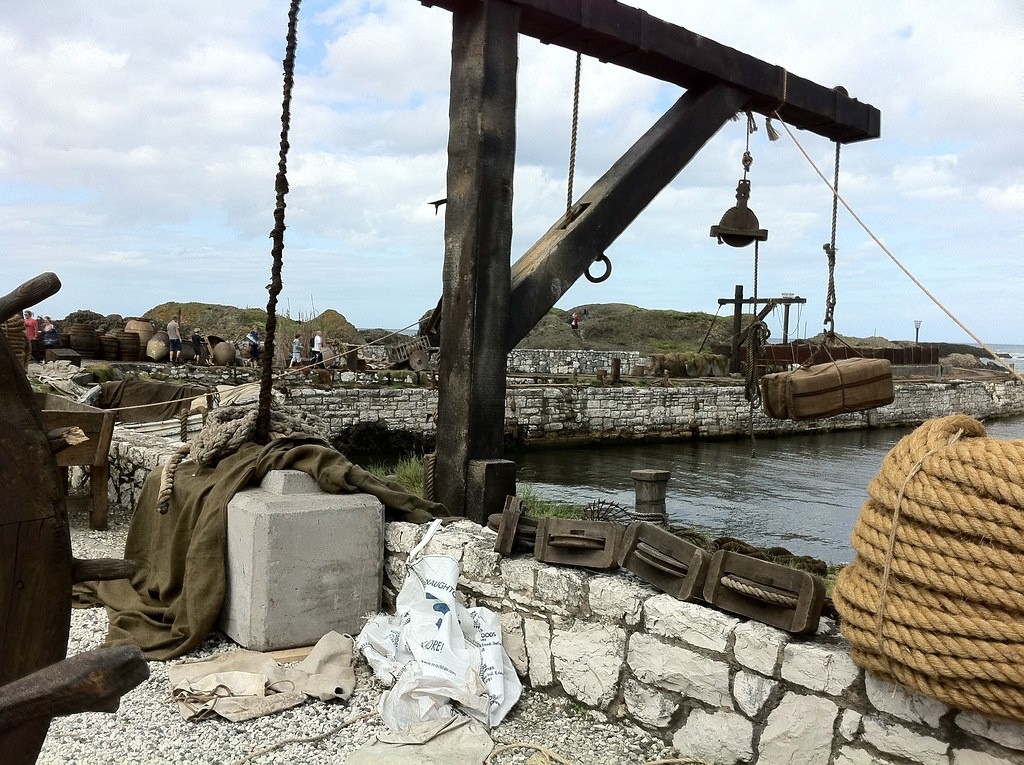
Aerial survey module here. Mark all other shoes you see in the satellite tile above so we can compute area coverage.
[175,357,180,363]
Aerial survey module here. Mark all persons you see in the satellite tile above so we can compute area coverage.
[39,316,60,351]
[24,310,38,359]
[245,324,260,368]
[166,316,183,364]
[567,313,579,329]
[190,327,204,363]
[309,330,330,369]
[289,332,304,368]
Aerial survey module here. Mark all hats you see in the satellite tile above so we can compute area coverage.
[194,328,200,332]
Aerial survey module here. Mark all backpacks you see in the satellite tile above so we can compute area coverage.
[310,337,314,347]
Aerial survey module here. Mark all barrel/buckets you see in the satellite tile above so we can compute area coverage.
[116,332,141,361]
[145,331,170,363]
[69,322,96,359]
[98,336,118,361]
[124,318,154,361]
[213,342,236,366]
